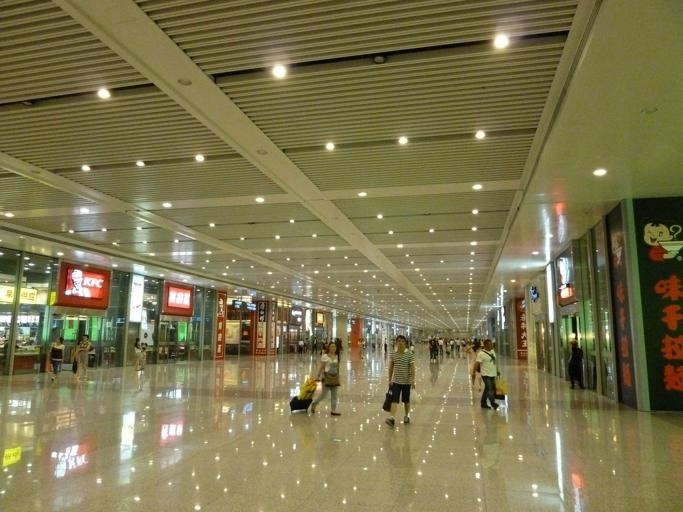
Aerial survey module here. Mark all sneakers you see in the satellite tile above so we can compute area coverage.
[404,417,409,423]
[385,417,394,427]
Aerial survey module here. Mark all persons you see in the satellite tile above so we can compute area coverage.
[310,341,341,416]
[567,341,585,389]
[308,416,338,484]
[473,409,506,495]
[46,337,64,383]
[65,269,91,299]
[382,428,416,508]
[73,334,91,380]
[290,336,485,365]
[139,347,146,370]
[384,334,415,428]
[133,337,141,371]
[471,338,500,409]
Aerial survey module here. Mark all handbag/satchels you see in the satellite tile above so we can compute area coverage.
[324,374,339,386]
[298,384,316,400]
[383,388,393,411]
[495,375,509,400]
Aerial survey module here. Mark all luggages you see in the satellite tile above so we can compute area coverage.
[290,380,320,412]
